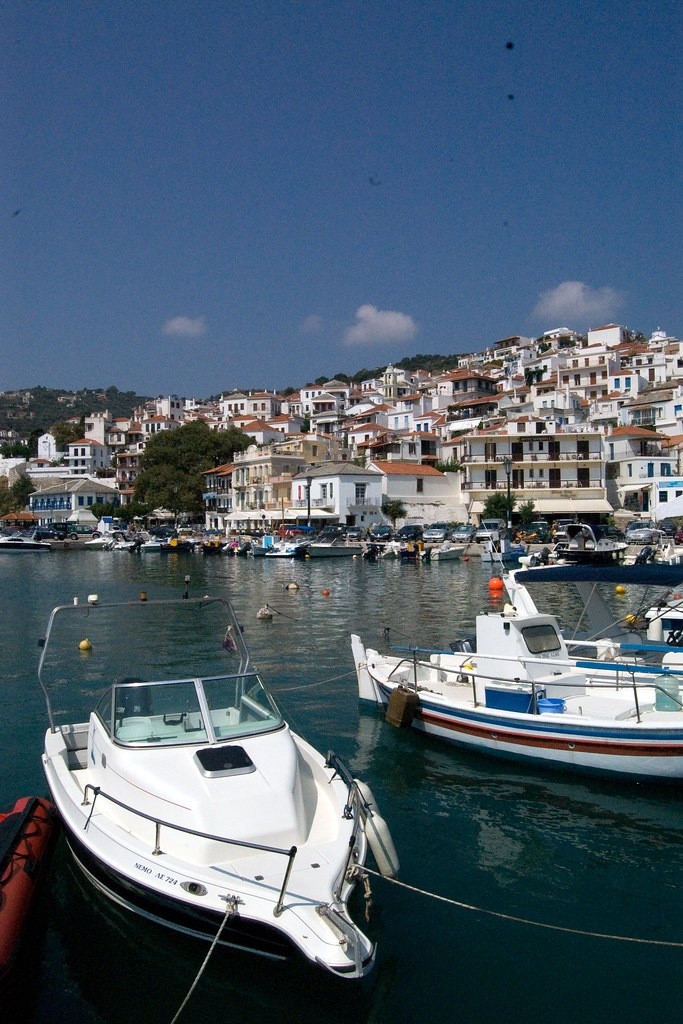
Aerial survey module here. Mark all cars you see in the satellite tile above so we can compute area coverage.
[421,522,454,543]
[369,524,395,542]
[394,525,424,542]
[342,525,368,542]
[452,524,477,543]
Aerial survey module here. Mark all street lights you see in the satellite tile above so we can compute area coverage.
[502,459,515,541]
[304,466,314,527]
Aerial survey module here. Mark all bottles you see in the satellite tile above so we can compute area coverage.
[656,667,681,711]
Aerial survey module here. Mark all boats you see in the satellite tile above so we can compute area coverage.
[348,563,683,788]
[0,518,683,574]
[643,583,683,650]
[37,595,398,981]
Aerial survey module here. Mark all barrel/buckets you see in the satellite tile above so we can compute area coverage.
[537,698,567,714]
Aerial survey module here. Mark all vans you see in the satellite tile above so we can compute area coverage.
[474,519,507,544]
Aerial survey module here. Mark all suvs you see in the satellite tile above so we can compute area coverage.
[29,525,66,542]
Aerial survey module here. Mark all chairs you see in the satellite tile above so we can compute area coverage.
[569,539,579,549]
[117,717,154,732]
[585,540,595,549]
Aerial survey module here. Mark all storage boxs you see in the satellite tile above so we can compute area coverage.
[484,680,546,714]
